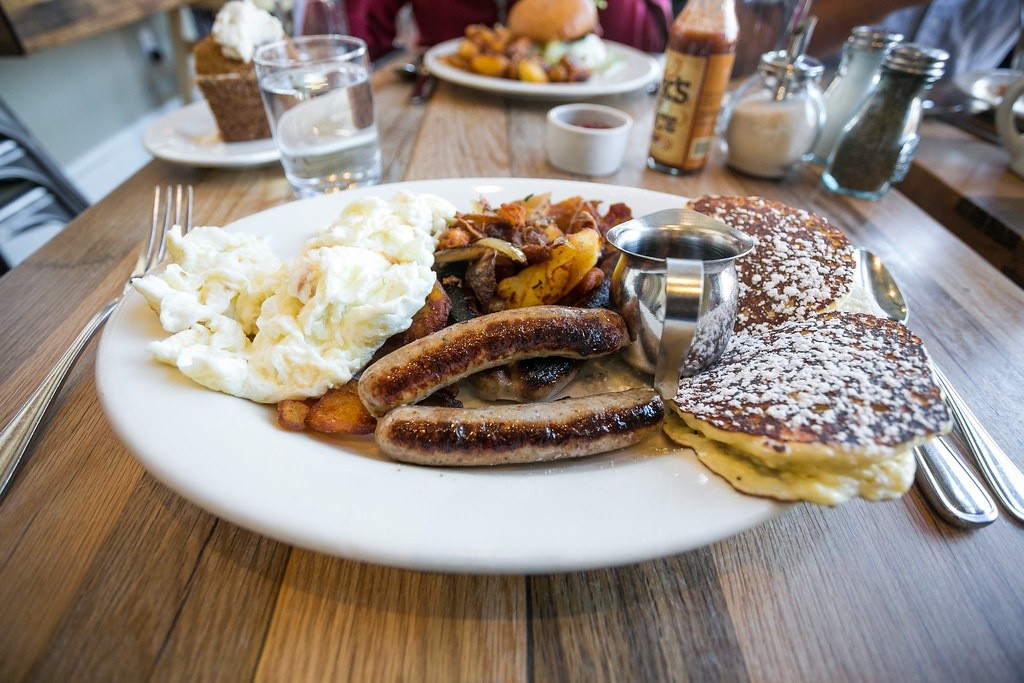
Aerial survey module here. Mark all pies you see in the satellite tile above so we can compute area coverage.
[661,194,954,507]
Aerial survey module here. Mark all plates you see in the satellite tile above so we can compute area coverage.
[140,99,281,169]
[91,176,885,577]
[421,34,660,100]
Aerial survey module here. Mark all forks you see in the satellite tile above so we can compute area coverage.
[0,183,195,507]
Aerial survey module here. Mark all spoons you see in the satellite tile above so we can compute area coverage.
[848,247,1024,523]
[392,54,426,79]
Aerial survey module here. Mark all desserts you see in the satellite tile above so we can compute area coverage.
[194,0,305,144]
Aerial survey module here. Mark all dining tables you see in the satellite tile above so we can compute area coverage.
[0,0,1024,683]
[901,94,1024,291]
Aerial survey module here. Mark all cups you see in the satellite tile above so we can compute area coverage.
[994,77,1024,178]
[603,206,754,401]
[248,0,350,80]
[543,103,633,178]
[250,32,383,200]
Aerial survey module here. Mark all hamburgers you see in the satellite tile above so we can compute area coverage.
[506,0,609,81]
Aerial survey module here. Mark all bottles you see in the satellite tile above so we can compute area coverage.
[719,49,827,180]
[820,41,949,203]
[811,24,905,169]
[645,0,742,177]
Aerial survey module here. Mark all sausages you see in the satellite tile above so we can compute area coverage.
[374,387,667,467]
[438,250,619,403]
[356,306,630,419]
